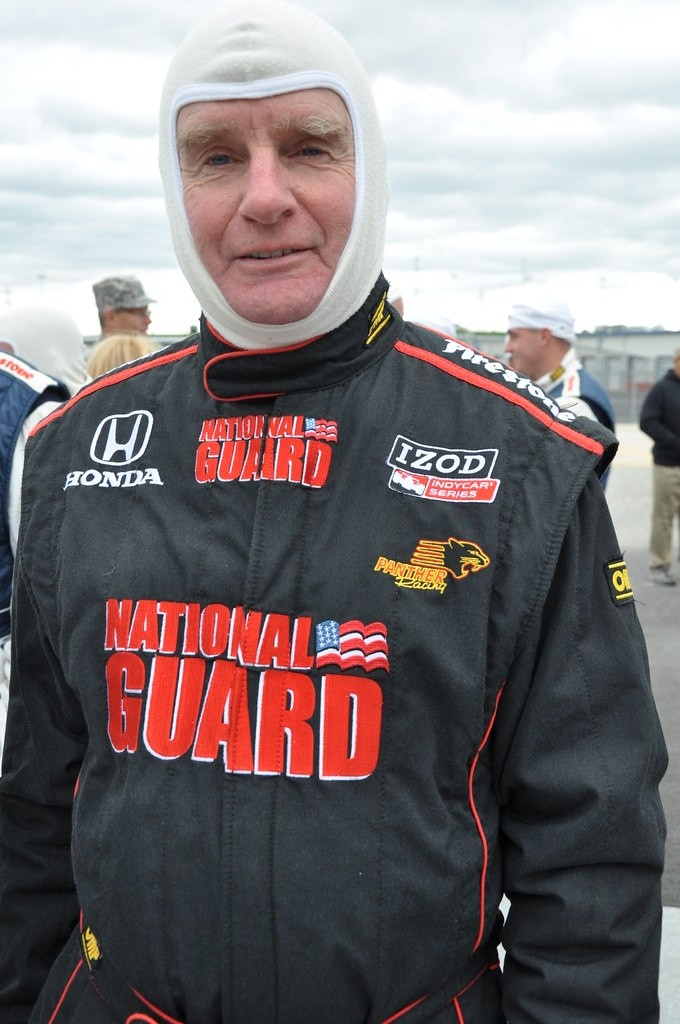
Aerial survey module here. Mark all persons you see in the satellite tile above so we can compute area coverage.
[504,301,615,491]
[0,350,73,747]
[640,348,680,585]
[0,2,670,1024]
[86,276,156,378]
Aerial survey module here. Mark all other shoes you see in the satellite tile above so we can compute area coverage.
[648,565,677,585]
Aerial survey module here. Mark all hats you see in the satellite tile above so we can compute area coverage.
[93,274,157,316]
[508,293,576,344]
